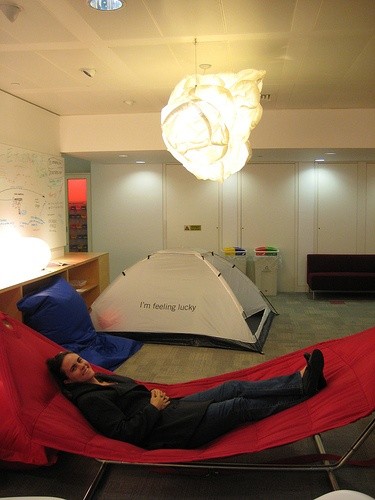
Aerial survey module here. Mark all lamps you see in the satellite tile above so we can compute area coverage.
[161,38,267,182]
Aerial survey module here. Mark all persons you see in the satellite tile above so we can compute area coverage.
[46,349,328,451]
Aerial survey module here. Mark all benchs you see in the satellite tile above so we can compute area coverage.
[307,254,375,301]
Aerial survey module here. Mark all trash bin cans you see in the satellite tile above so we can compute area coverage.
[254,246,279,296]
[221,247,246,275]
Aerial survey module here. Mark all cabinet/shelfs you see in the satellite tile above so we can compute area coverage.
[0,253,110,323]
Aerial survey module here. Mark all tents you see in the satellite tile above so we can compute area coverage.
[86,247,279,357]
